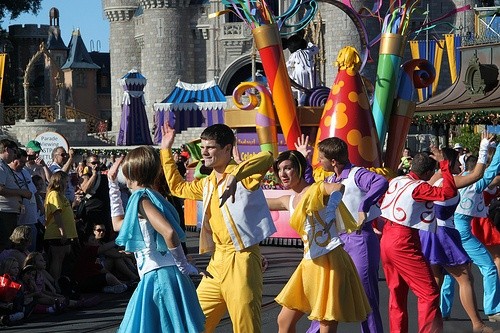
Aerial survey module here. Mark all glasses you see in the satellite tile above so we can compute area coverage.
[95,229,106,232]
[9,147,17,154]
[56,153,67,157]
[89,162,99,165]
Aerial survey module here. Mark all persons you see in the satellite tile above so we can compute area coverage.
[106,145,214,333]
[294,134,389,333]
[371,128,500,333]
[266,149,373,333]
[159,121,277,333]
[0,137,190,321]
[397,147,412,177]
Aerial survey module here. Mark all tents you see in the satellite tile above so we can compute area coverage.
[152,79,227,145]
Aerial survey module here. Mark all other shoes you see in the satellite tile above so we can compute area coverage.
[104,284,125,293]
[79,295,99,309]
[488,313,500,323]
[53,299,63,314]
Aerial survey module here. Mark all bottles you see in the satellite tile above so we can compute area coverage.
[103,284,129,293]
[1,311,27,323]
[47,303,61,312]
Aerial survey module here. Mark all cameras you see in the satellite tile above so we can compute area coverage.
[95,163,107,171]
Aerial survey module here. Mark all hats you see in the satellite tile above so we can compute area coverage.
[26,140,44,153]
[453,143,463,149]
